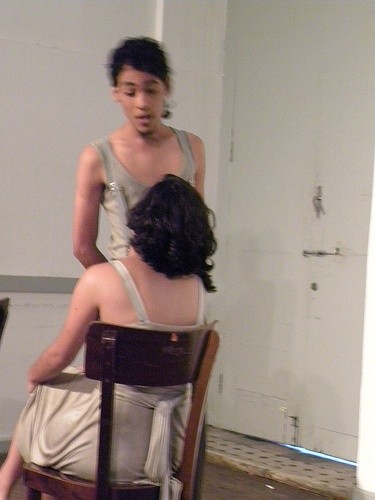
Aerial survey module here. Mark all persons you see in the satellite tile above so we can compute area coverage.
[72,36,207,270]
[0,174,218,500]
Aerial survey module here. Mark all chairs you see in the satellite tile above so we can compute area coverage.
[21,319,221,500]
